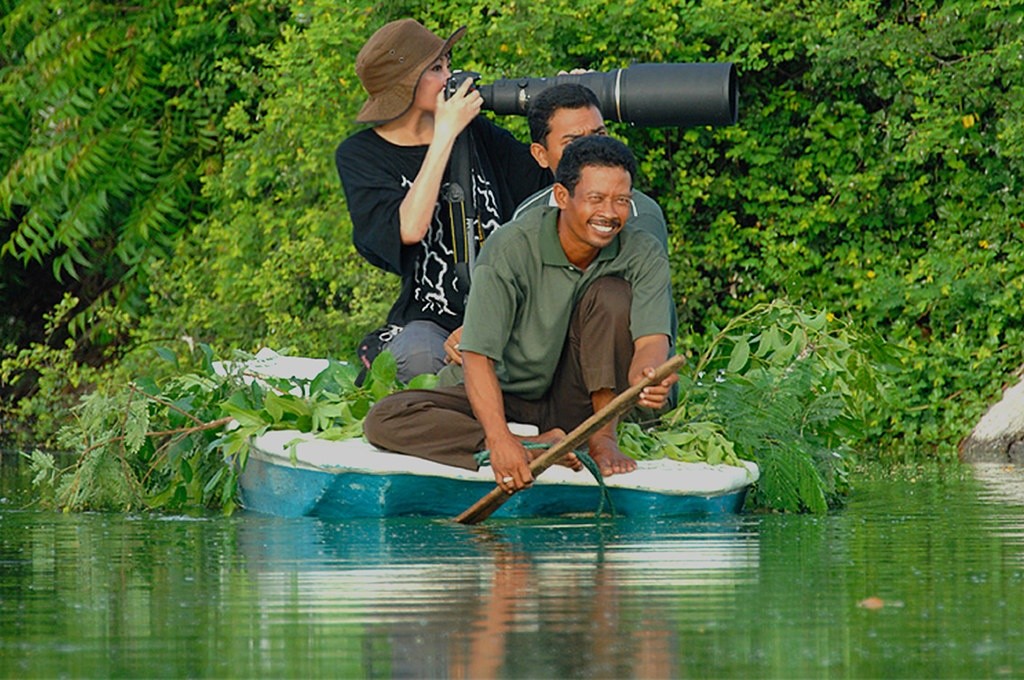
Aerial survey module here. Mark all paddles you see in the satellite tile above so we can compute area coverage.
[455,352,688,525]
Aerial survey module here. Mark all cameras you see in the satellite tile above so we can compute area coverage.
[444,62,742,128]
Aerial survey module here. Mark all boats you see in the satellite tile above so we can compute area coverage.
[211,347,761,521]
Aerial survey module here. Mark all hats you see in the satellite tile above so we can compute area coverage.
[352,19,467,123]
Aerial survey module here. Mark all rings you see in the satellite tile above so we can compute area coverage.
[502,477,513,483]
[452,344,460,351]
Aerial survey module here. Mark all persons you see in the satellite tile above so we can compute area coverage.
[336,19,596,388]
[363,134,678,496]
[436,83,678,423]
